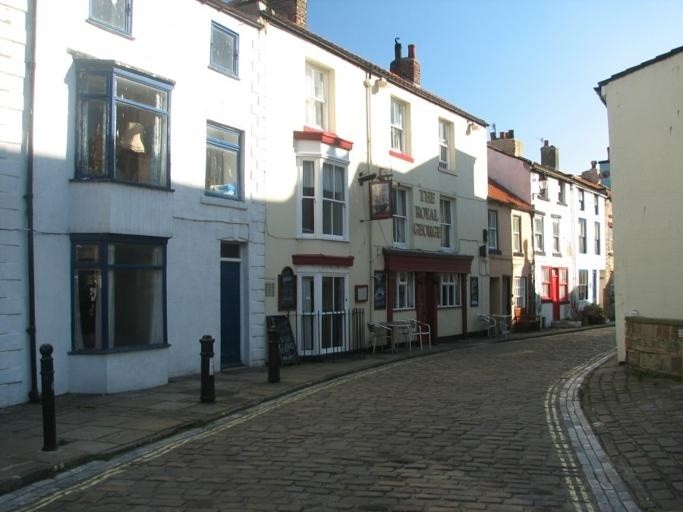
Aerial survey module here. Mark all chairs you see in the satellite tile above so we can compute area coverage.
[478,313,497,337]
[369,319,431,353]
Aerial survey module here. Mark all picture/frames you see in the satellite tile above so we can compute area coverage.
[368,178,393,220]
[355,285,368,302]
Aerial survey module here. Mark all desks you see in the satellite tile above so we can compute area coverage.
[515,315,541,331]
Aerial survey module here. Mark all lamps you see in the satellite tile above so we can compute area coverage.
[470,123,479,130]
[378,77,390,88]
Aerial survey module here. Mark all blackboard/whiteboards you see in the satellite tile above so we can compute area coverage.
[266,315,299,365]
